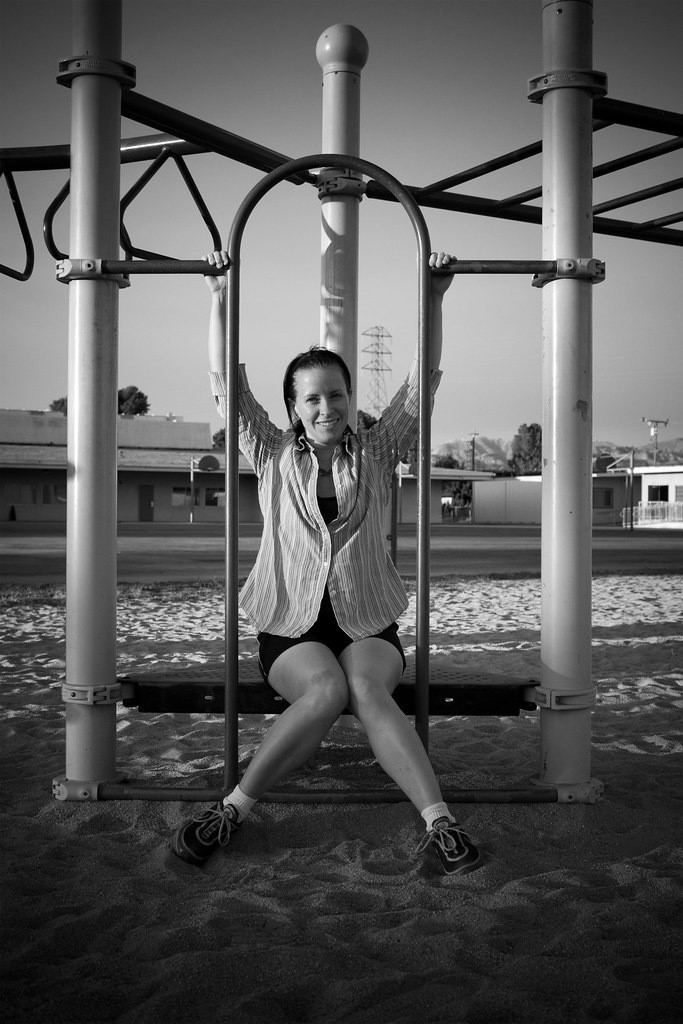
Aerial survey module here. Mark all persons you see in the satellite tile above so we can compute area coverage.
[173,251,484,876]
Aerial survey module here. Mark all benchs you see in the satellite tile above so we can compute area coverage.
[122,662,541,720]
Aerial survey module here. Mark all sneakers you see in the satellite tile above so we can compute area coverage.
[172,800,246,863]
[416,817,480,875]
